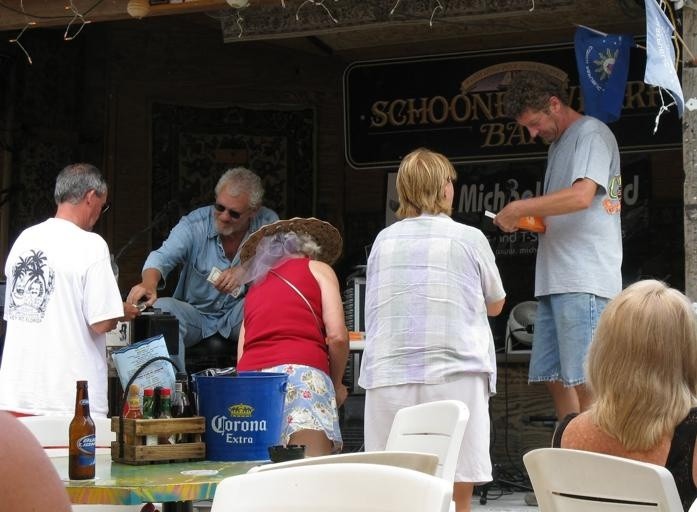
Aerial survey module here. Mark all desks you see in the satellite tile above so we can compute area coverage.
[36,457,278,512]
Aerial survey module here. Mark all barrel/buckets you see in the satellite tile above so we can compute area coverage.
[190,369,289,463]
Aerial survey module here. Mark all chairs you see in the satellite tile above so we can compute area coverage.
[20,418,112,455]
[212,464,450,512]
[383,401,470,512]
[245,450,439,474]
[524,447,682,512]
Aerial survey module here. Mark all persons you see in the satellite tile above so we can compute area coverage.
[356,149,507,511]
[126,167,281,373]
[494,71,623,419]
[0,164,126,420]
[235,217,350,461]
[552,278,697,508]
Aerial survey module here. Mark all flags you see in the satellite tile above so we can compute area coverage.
[571,19,637,128]
[643,0,691,122]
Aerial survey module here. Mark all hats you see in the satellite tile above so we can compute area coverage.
[240,217,343,270]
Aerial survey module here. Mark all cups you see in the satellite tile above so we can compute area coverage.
[268,444,307,465]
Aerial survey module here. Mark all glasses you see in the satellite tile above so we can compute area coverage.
[215,203,253,218]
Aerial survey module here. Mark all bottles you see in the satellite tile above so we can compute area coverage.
[176,372,194,443]
[68,381,96,479]
[124,386,145,446]
[141,389,153,419]
[158,389,175,444]
[172,383,192,443]
[146,387,164,445]
